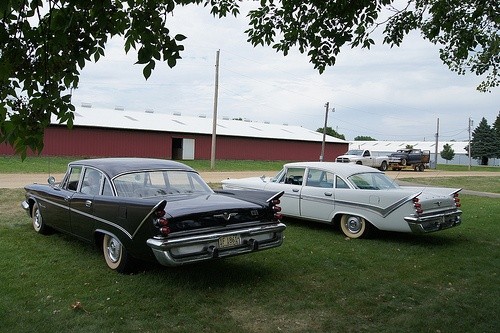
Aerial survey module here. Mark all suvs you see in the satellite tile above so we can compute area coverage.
[388,148,430,171]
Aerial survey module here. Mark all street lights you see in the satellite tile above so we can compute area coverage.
[319,108,336,161]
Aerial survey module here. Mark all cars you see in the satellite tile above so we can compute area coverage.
[21,158,287,274]
[220,162,464,239]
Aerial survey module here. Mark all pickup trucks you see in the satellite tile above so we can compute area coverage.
[335,149,389,170]
